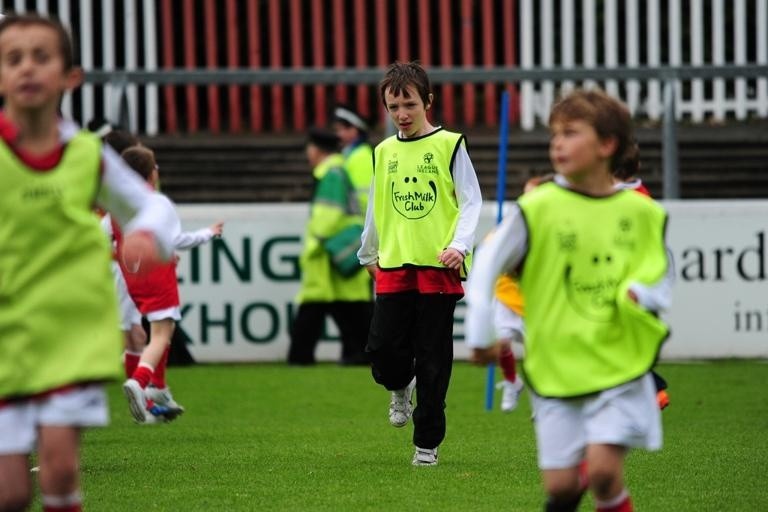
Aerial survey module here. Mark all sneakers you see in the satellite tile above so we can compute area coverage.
[123,378,184,424]
[500,376,524,412]
[655,389,670,410]
[389,375,417,427]
[413,446,438,466]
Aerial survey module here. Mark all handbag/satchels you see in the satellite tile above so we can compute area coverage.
[327,224,364,276]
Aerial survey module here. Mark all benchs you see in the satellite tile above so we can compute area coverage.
[144,118,765,205]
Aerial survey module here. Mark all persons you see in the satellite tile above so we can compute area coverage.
[1,17,180,512]
[465,90,669,512]
[85,116,224,426]
[287,106,373,364]
[357,64,482,465]
[483,141,669,411]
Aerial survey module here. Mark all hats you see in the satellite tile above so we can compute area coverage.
[333,103,373,133]
